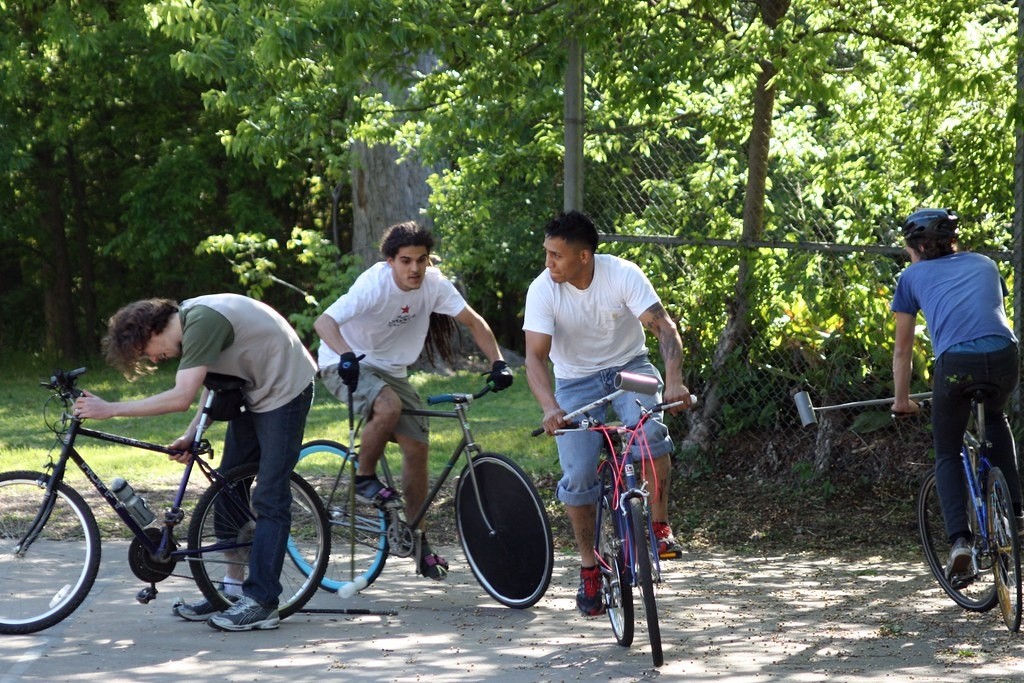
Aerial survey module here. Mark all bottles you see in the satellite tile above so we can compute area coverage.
[110,477,157,528]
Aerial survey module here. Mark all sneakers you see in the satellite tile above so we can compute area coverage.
[397,530,448,580]
[173,583,240,620]
[1016,511,1024,536]
[207,594,280,630]
[944,537,972,580]
[576,565,606,615]
[353,477,403,508]
[652,518,682,558]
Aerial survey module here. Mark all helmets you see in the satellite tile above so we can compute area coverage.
[902,209,959,239]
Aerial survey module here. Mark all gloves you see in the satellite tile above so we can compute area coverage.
[480,361,513,393]
[338,353,365,393]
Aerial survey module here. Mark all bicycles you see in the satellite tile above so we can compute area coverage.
[0,366,331,634]
[891,383,1022,632]
[555,395,698,667]
[287,360,554,611]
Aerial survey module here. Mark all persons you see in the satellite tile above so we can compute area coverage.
[313,221,514,581]
[524,212,692,616]
[890,210,1024,582]
[69,292,318,632]
